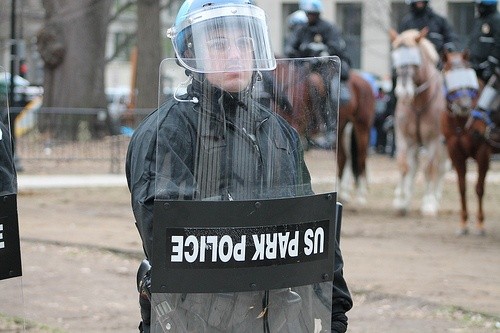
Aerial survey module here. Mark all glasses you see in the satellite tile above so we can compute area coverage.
[203,37,255,54]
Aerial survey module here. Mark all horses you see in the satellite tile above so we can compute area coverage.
[270,27,500,237]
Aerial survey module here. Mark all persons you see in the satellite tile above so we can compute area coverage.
[284,0,500,155]
[125,0,353,333]
[0,67,45,196]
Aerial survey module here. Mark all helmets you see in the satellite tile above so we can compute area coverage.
[166,0,276,73]
[287,10,309,30]
[477,0,496,5]
[407,0,429,5]
[300,0,321,13]
